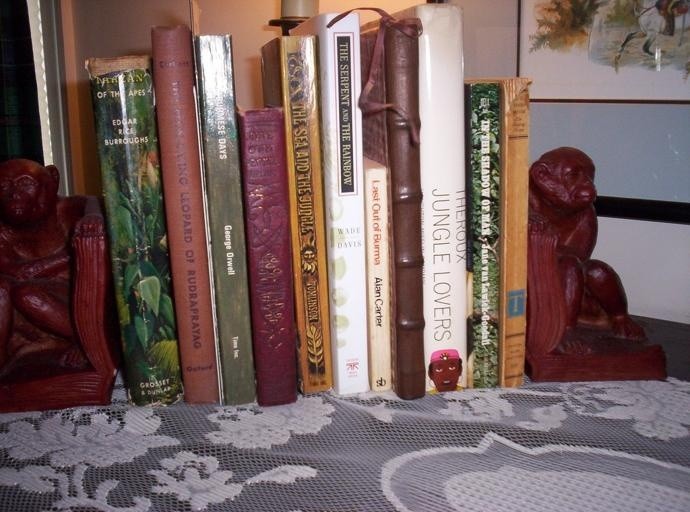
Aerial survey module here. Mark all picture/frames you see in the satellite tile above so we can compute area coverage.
[516,0,690,103]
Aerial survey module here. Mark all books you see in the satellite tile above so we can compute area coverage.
[290,12,369,395]
[83,54,180,408]
[260,35,333,395]
[464,76,532,389]
[359,18,426,400]
[465,82,500,387]
[360,4,467,391]
[151,22,218,404]
[237,105,298,407]
[191,32,255,406]
[363,156,391,393]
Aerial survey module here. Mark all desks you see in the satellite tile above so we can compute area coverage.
[0,376,690,512]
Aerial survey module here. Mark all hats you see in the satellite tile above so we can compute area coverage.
[430,348,460,363]
